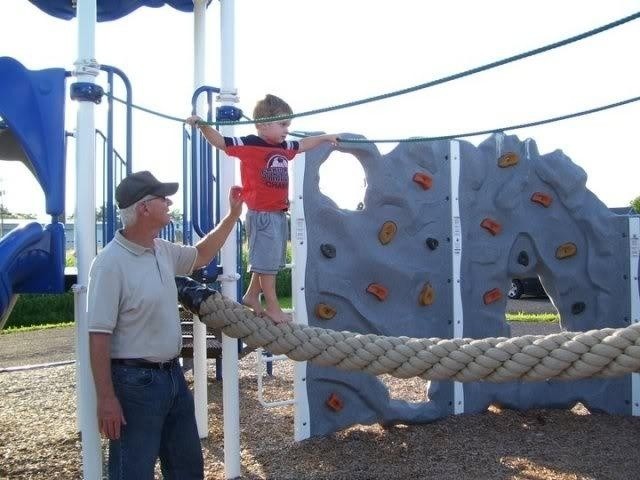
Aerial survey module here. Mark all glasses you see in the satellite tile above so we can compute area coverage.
[143,194,167,203]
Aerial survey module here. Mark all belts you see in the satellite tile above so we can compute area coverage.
[115,355,182,371]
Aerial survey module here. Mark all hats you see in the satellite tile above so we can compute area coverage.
[115,170,179,209]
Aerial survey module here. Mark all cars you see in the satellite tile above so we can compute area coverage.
[507,277,549,299]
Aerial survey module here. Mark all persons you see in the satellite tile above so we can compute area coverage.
[186,95,339,324]
[86,171,244,480]
